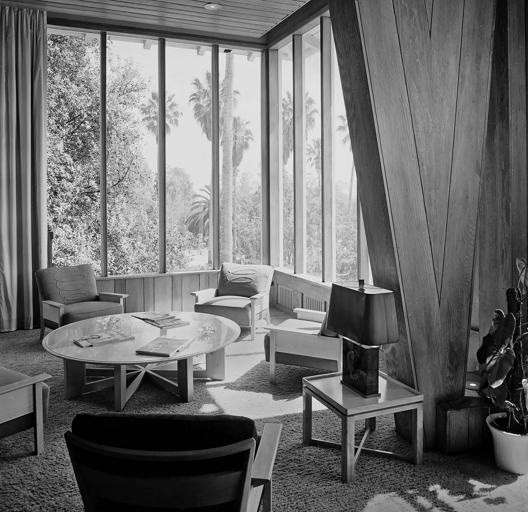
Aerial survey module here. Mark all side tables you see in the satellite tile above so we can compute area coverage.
[302,371,424,481]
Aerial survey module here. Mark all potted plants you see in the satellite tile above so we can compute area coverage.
[477,257,528,476]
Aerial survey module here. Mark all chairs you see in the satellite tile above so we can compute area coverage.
[191,262,275,340]
[0,367,51,457]
[65,412,283,510]
[34,264,128,337]
[263,306,341,383]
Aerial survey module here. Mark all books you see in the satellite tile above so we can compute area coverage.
[149,315,175,321]
[74,328,135,348]
[155,317,182,323]
[136,336,191,357]
[147,321,190,328]
[131,310,168,318]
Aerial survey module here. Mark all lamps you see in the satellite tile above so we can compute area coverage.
[328,280,400,398]
